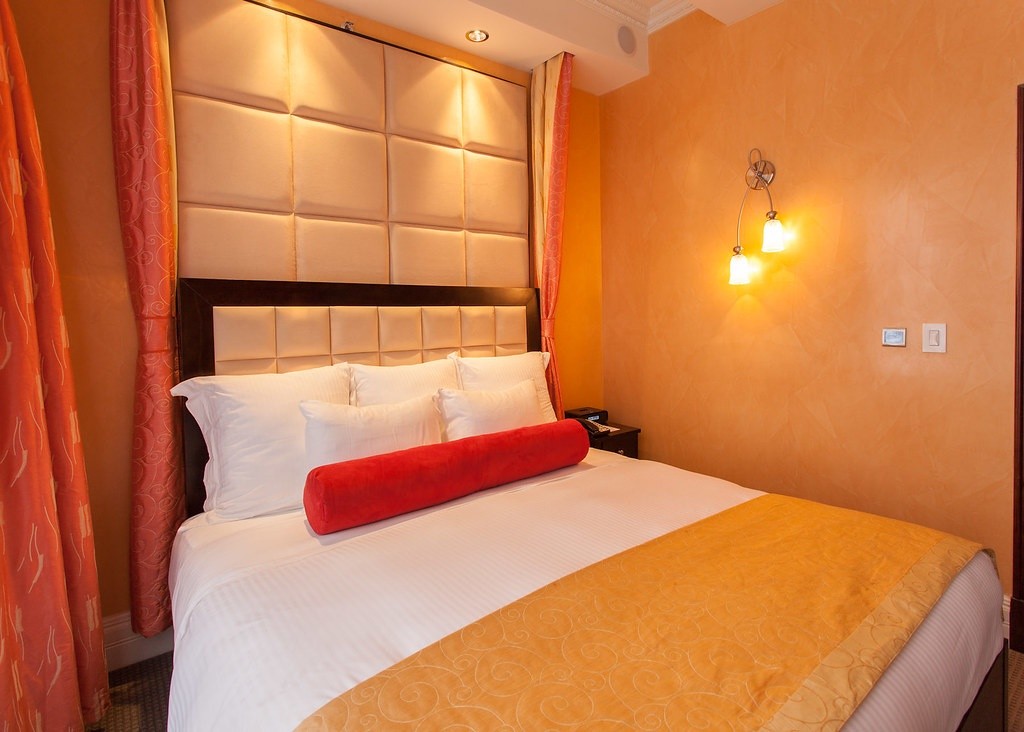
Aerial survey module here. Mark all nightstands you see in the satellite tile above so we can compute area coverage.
[583,421,641,458]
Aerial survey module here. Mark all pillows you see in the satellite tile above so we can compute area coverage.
[304,419,590,536]
[171,360,351,522]
[436,380,536,442]
[339,350,458,425]
[300,387,441,472]
[448,351,558,425]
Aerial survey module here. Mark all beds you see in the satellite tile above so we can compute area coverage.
[165,275,1007,732]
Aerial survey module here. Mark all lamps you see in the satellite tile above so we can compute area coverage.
[730,147,792,284]
